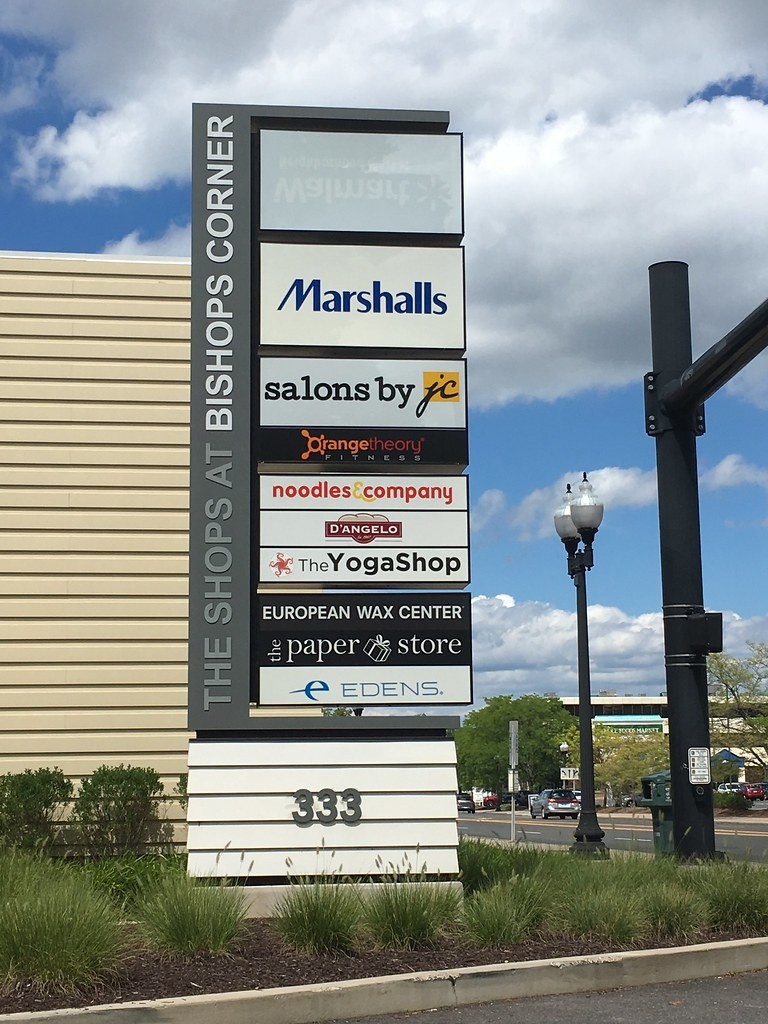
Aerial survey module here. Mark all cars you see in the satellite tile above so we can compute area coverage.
[530,789,580,819]
[483,794,498,807]
[621,794,641,807]
[718,782,768,801]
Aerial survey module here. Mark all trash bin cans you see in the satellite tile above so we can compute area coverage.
[642,769,673,854]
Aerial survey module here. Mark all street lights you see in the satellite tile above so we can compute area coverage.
[553,473,611,860]
[559,742,569,789]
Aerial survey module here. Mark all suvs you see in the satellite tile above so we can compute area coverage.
[457,793,475,814]
